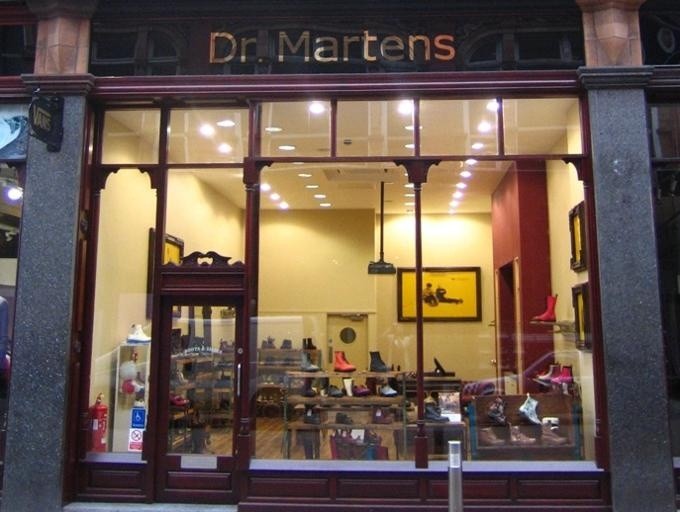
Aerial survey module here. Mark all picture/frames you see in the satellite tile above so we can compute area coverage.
[397,267,483,322]
[567,200,594,353]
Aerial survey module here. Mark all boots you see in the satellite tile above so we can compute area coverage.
[369,350,391,372]
[298,350,322,371]
[298,378,399,397]
[334,350,356,371]
[536,361,575,387]
[479,426,506,446]
[518,396,542,425]
[509,425,537,445]
[530,295,557,322]
[539,422,568,445]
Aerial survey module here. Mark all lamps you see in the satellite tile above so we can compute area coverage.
[368,182,397,275]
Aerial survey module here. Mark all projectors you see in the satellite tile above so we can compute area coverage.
[368,263,396,274]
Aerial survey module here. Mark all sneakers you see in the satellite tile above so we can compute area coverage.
[260,335,317,349]
[406,401,450,423]
[487,395,508,427]
[301,405,394,424]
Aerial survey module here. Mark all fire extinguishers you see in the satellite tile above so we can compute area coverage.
[89,392,109,452]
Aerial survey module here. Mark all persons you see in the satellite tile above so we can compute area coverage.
[422,282,437,306]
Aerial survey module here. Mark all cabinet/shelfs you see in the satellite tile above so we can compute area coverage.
[284,370,584,461]
[168,345,322,454]
